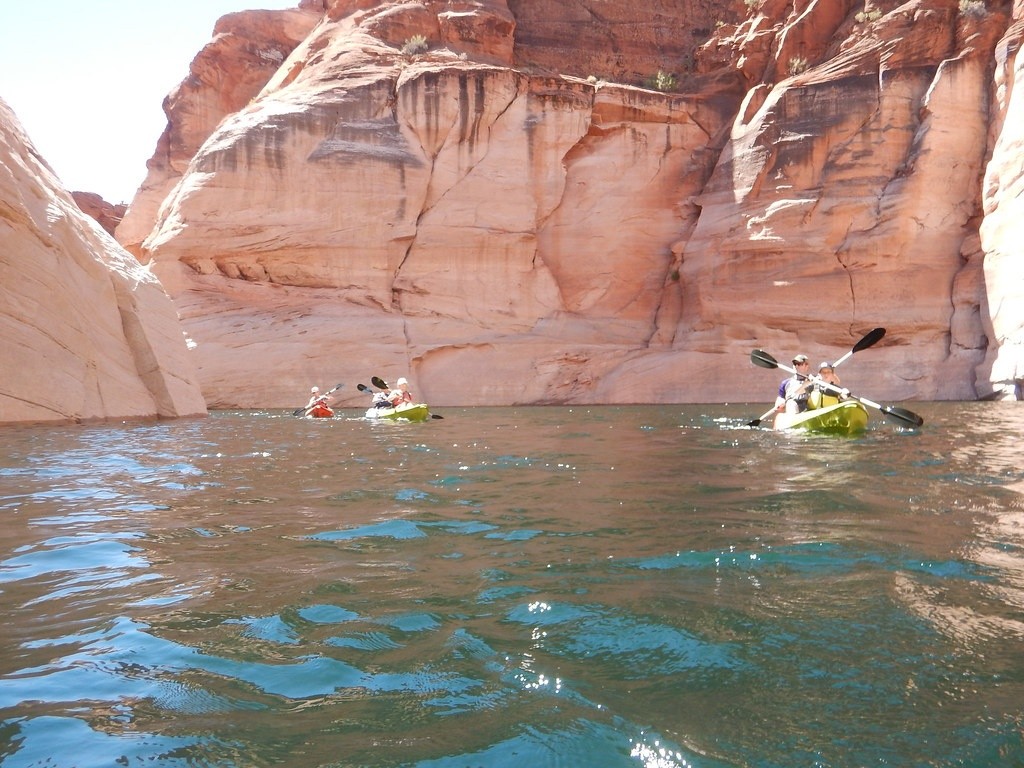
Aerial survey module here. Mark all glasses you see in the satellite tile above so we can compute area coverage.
[793,362,802,366]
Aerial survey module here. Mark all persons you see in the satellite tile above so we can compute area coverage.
[372,378,412,408]
[307,386,331,409]
[775,355,840,414]
[807,362,851,409]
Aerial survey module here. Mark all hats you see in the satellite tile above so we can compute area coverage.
[397,378,407,387]
[310,387,319,393]
[818,362,832,373]
[792,355,809,362]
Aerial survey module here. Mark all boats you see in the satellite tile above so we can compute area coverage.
[773,400,870,438]
[364,402,428,423]
[304,404,335,419]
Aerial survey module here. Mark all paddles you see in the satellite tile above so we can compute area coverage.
[357,383,376,397]
[371,375,443,420]
[750,347,922,431]
[745,327,887,429]
[291,382,345,418]
